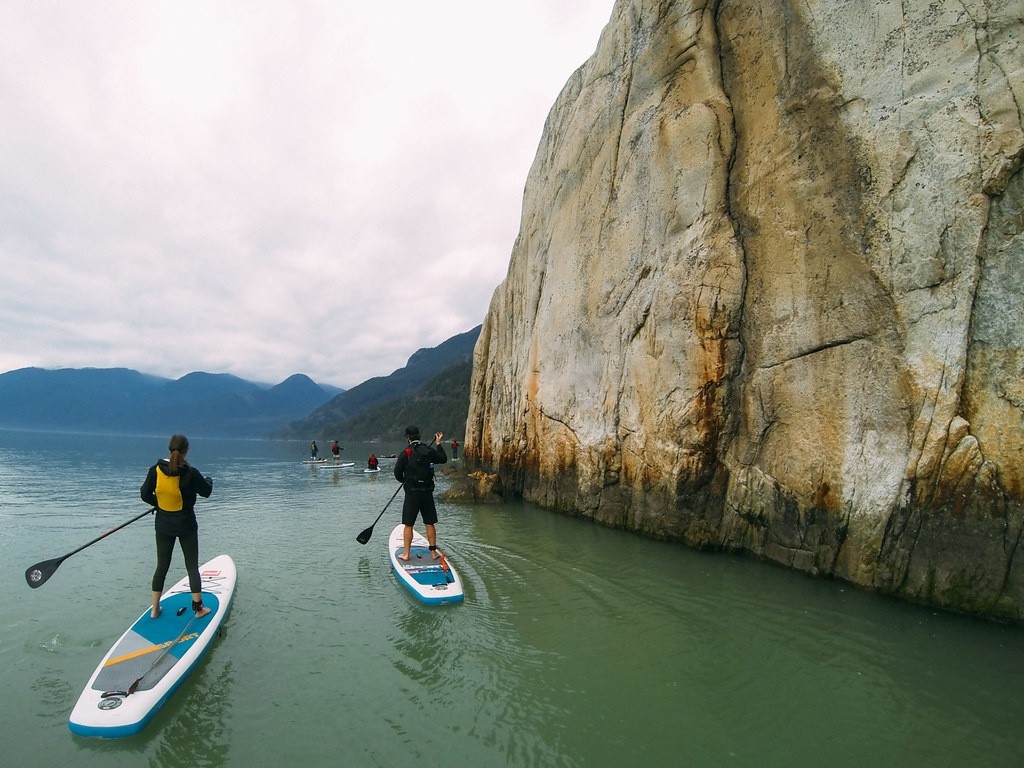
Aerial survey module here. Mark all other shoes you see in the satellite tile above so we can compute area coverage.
[454,457,458,459]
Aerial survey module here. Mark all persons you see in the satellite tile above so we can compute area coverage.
[393,426,448,561]
[332,441,344,466]
[140,435,213,617]
[368,454,379,470]
[451,439,460,458]
[311,441,318,460]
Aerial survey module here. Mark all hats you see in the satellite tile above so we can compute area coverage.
[402,426,419,437]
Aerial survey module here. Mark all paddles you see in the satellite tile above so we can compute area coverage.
[23,501,158,590]
[355,437,439,545]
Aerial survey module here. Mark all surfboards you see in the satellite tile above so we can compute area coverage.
[66,554,240,737]
[303,460,328,463]
[363,466,381,472]
[320,463,354,468]
[387,523,465,606]
